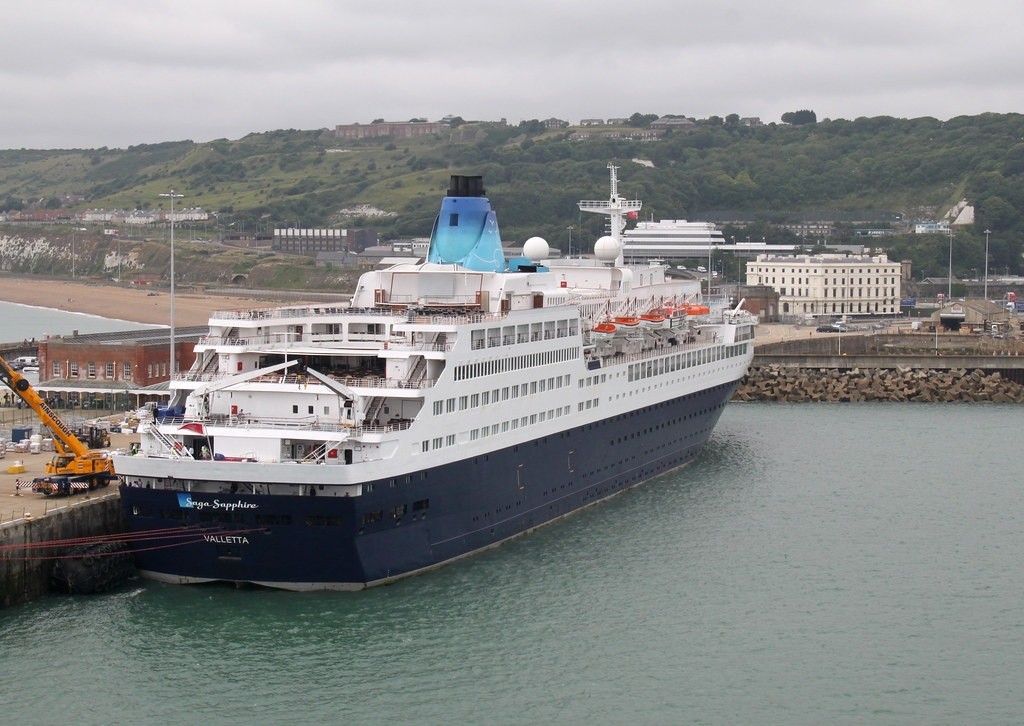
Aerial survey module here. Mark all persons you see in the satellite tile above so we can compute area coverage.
[183,445,196,459]
[153,406,160,421]
[24,337,35,347]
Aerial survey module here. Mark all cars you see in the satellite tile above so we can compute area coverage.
[8,356,39,371]
[816,315,893,332]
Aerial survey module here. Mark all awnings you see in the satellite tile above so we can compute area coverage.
[32,379,142,412]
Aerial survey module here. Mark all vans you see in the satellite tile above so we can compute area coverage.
[677,266,686,270]
[697,266,707,272]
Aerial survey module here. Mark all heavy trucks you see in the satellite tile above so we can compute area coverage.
[1006,300,1024,311]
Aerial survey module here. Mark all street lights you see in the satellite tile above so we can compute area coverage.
[983,230,991,299]
[948,230,956,300]
[566,226,573,258]
[159,190,184,399]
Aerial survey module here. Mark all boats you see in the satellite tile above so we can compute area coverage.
[112,163,758,590]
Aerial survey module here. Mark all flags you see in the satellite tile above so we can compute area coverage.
[177,423,203,433]
[628,211,638,220]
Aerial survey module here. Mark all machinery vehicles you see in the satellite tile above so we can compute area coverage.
[87,427,110,448]
[0,356,115,497]
